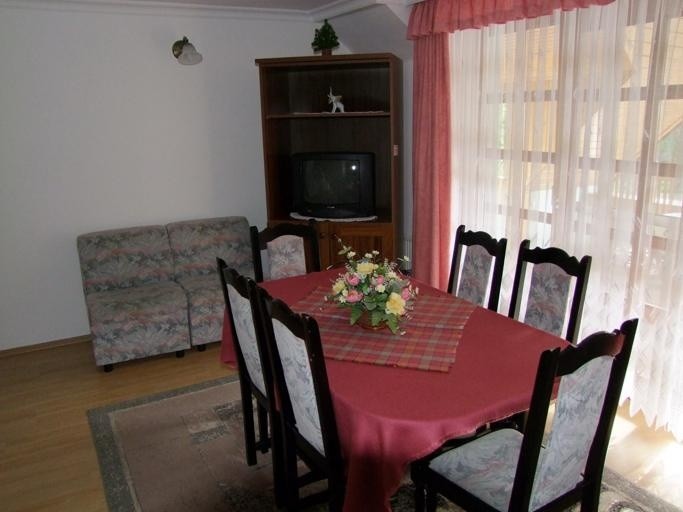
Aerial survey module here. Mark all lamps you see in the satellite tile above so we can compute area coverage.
[171,35,203,66]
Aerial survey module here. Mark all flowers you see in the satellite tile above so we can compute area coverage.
[320,229,419,336]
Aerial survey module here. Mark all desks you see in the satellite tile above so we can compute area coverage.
[220,261,587,512]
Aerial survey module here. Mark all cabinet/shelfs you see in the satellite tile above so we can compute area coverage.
[253,51,405,274]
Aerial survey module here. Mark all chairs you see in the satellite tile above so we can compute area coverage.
[215,254,285,512]
[507,237,592,346]
[248,282,346,512]
[166,214,256,353]
[408,314,640,512]
[250,216,322,284]
[446,224,508,314]
[76,224,191,374]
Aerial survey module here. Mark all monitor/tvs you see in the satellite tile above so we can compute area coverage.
[288,151,379,223]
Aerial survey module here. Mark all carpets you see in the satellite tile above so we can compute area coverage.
[84,372,682,512]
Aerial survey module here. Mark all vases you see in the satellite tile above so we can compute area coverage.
[355,309,386,331]
